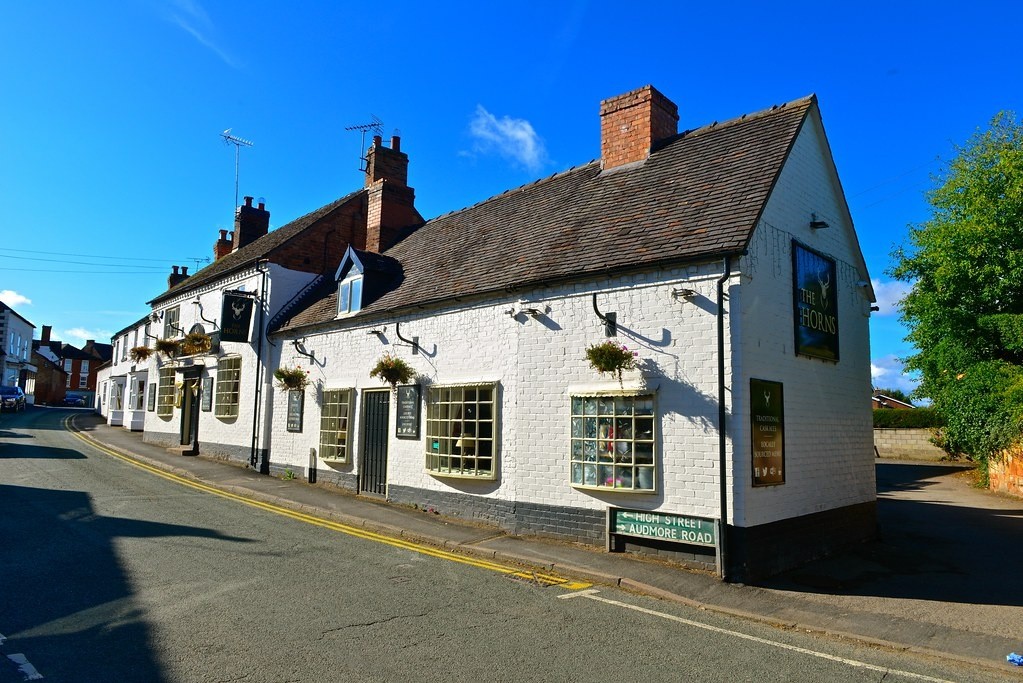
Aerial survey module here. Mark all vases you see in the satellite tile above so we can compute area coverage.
[599,366,616,370]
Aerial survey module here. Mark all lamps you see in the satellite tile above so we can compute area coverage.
[191,299,201,305]
[671,288,694,296]
[366,330,381,335]
[811,221,830,230]
[289,341,300,345]
[148,312,158,322]
[456,434,475,469]
[520,309,540,314]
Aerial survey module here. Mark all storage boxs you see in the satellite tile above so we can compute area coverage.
[606,477,623,488]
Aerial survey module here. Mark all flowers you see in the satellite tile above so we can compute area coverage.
[584,341,638,391]
[274,366,311,393]
[369,353,416,392]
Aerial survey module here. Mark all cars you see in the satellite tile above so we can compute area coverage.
[63,393,87,407]
[0,386,26,413]
